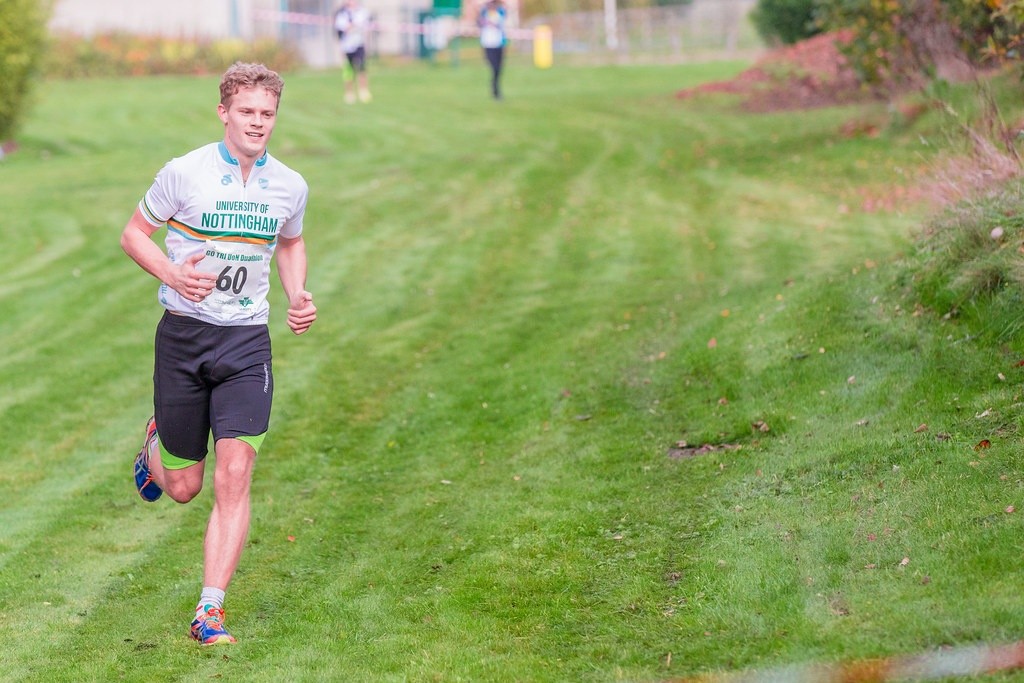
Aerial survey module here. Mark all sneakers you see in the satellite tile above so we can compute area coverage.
[133,415,163,503]
[189,606,239,645]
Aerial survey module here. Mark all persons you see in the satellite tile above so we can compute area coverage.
[476,0,511,98]
[120,58,316,646]
[334,0,373,105]
